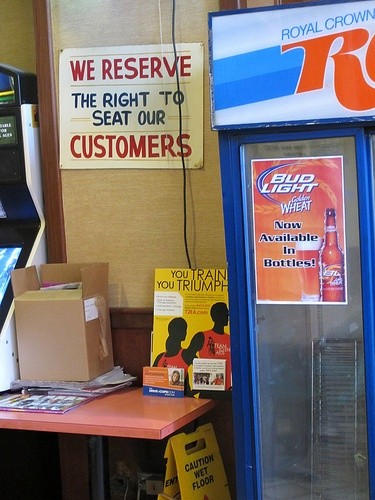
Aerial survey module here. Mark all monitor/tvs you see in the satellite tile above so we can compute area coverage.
[1,246,22,305]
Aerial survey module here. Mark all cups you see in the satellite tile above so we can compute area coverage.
[296,237,324,302]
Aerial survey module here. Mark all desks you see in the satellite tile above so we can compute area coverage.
[0,384,216,500]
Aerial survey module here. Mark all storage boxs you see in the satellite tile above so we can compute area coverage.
[8,261,115,384]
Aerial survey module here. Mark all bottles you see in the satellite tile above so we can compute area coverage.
[318,208,345,302]
